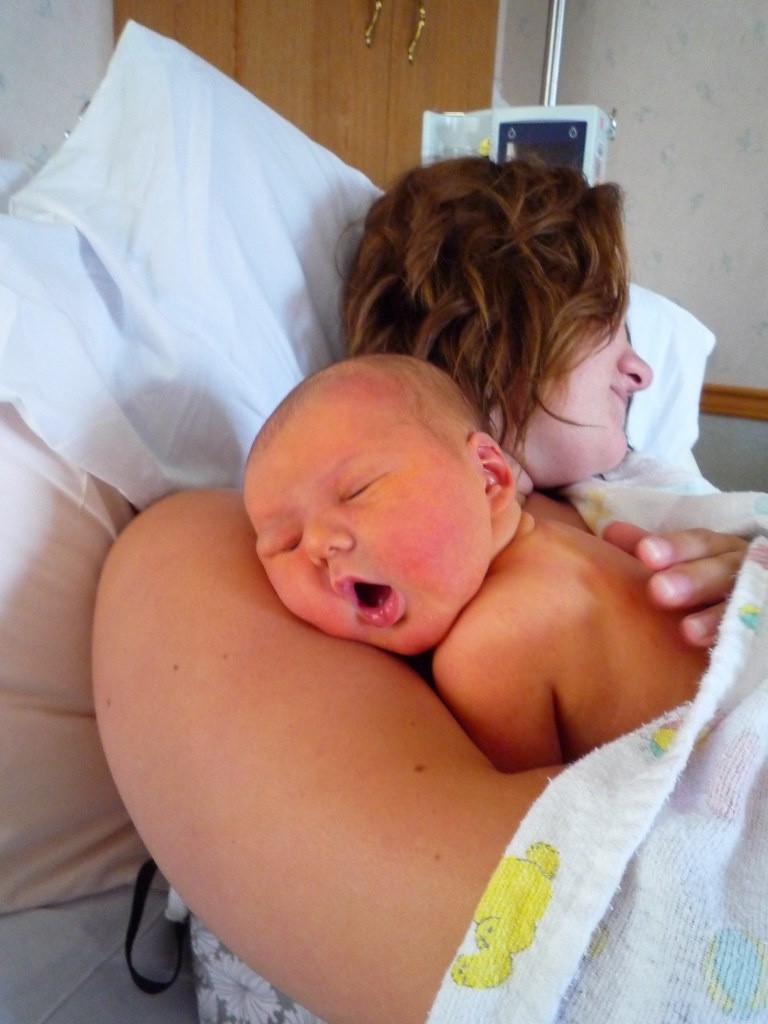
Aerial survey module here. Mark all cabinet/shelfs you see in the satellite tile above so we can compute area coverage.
[112,0,500,203]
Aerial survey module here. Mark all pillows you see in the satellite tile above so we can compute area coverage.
[1,17,716,541]
[0,163,165,914]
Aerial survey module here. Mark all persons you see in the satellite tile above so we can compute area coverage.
[243,354,716,774]
[90,154,767,1024]
